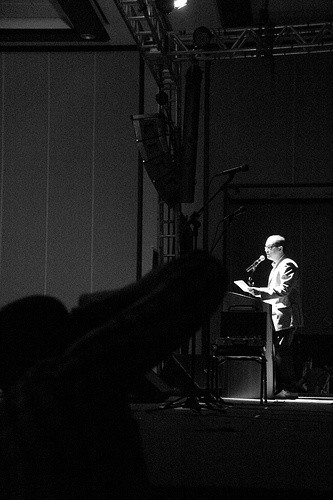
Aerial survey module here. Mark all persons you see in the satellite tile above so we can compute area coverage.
[248,234,305,399]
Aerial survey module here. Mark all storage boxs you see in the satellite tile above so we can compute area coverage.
[212,305,267,355]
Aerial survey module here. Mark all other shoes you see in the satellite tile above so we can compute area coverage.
[272,388,298,399]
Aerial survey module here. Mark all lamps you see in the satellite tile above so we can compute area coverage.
[193,26,213,48]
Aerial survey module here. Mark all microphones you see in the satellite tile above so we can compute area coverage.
[215,164,249,177]
[224,206,246,220]
[246,255,265,272]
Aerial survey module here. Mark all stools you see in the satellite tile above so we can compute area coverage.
[211,355,268,406]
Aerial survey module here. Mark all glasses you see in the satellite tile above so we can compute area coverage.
[264,245,275,250]
